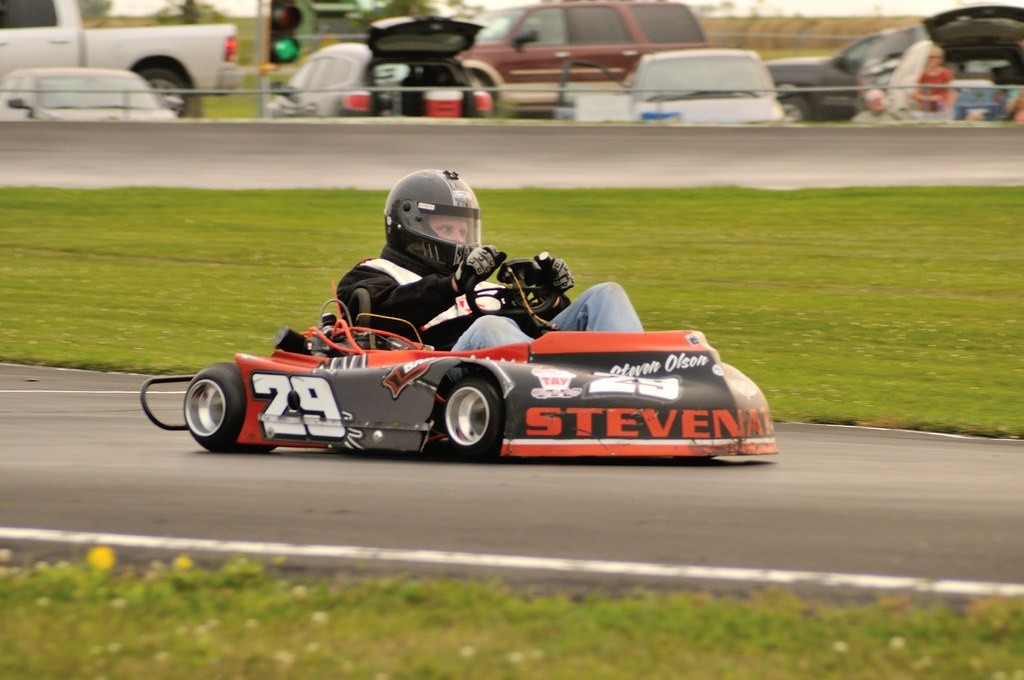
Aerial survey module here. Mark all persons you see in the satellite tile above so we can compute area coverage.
[336,166,645,354]
[851,44,1024,121]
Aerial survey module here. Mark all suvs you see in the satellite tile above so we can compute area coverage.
[267,15,496,122]
[449,1,710,118]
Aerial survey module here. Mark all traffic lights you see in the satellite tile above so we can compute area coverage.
[270,0,303,64]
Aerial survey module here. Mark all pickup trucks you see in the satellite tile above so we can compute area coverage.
[0,0,243,117]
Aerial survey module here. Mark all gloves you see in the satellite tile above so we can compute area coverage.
[451,242,509,292]
[536,250,578,296]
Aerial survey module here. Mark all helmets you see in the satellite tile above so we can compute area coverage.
[383,170,485,271]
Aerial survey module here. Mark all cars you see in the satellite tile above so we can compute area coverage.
[764,24,922,123]
[552,47,788,122]
[1,67,176,128]
